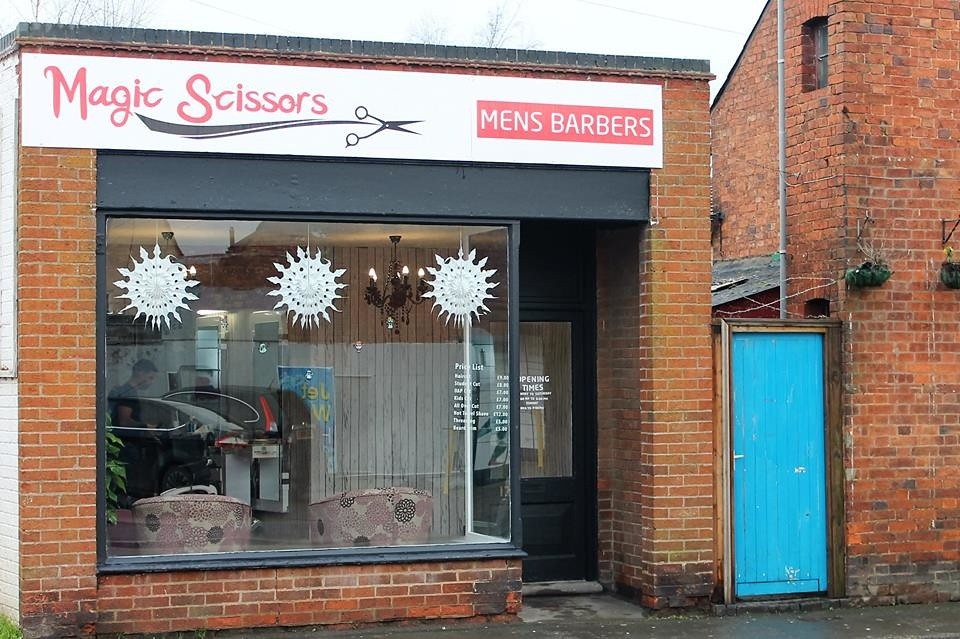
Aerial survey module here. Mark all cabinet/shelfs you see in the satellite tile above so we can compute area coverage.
[223,440,289,513]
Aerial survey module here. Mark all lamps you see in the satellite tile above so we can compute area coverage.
[362,236,429,335]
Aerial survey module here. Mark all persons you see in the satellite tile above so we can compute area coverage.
[108,357,162,506]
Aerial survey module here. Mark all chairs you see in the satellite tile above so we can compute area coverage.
[130,493,253,553]
[307,486,433,548]
